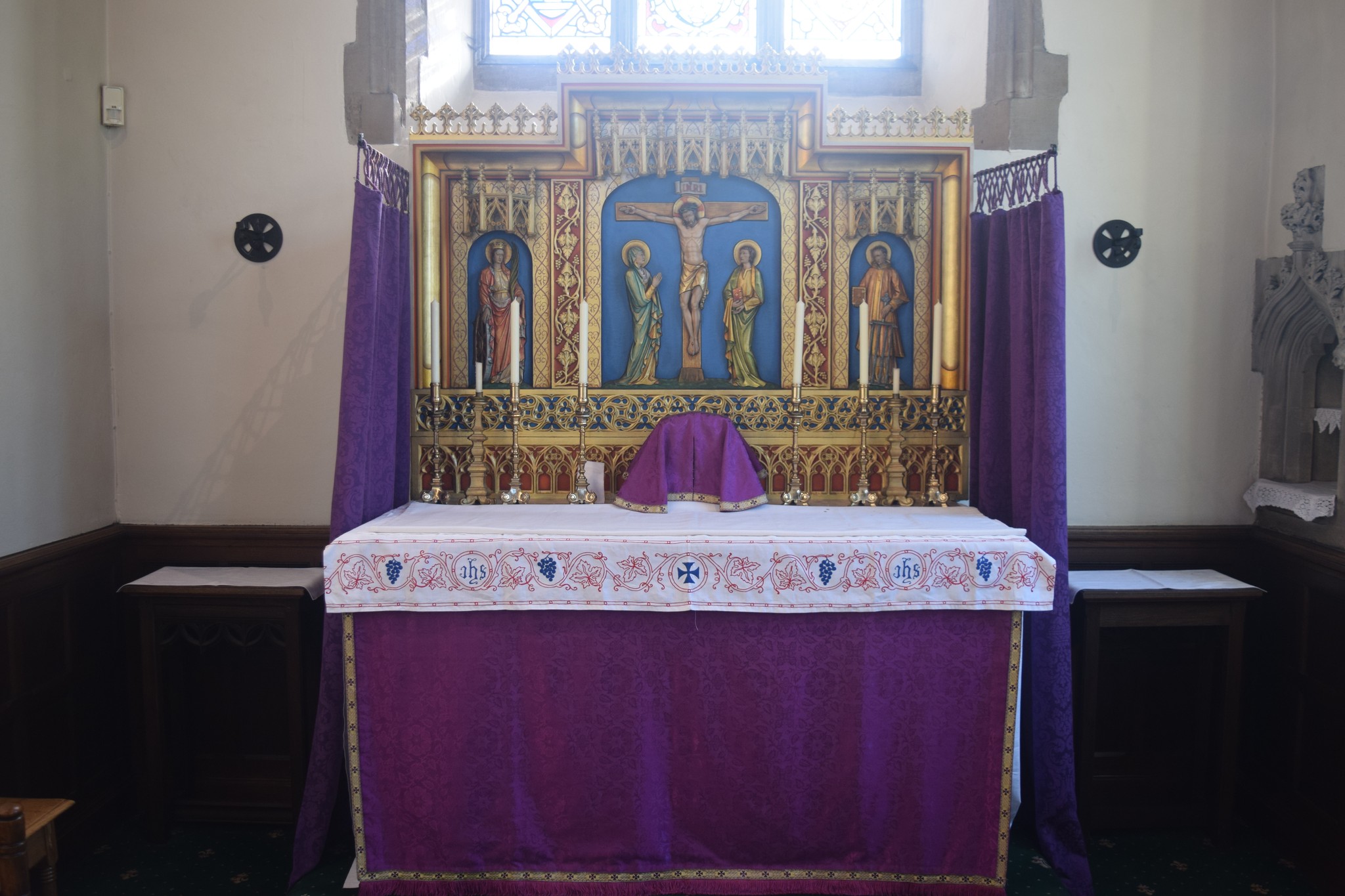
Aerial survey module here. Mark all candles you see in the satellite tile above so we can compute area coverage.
[858,298,870,385]
[930,296,944,386]
[475,361,483,393]
[509,296,521,384]
[578,297,589,384]
[792,296,805,385]
[892,366,901,395]
[430,296,441,384]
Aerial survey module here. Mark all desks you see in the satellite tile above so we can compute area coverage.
[319,498,1061,896]
[1066,555,1271,836]
[0,794,80,896]
[111,559,314,830]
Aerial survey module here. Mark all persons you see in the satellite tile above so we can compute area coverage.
[615,246,663,385]
[618,201,767,356]
[721,246,767,388]
[855,245,911,388]
[479,248,525,385]
